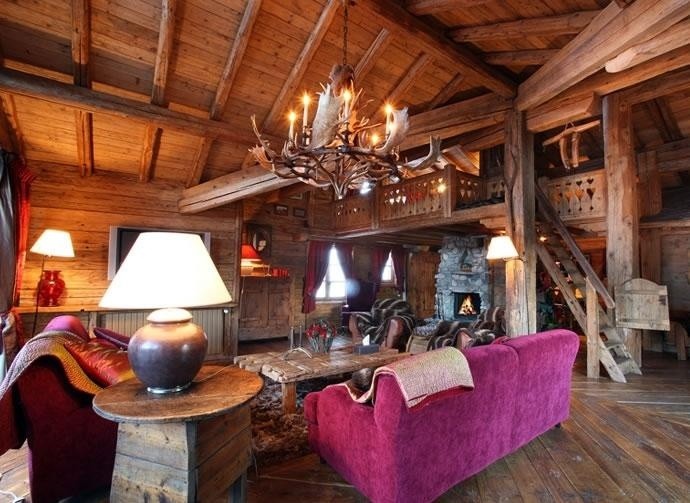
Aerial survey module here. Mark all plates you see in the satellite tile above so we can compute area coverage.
[238,275,292,341]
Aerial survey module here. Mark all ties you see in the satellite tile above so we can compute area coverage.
[107,226,211,280]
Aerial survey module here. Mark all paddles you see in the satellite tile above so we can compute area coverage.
[245,370,354,481]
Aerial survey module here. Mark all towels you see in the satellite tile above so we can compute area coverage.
[296,377,328,399]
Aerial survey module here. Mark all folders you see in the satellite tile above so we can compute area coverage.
[100,308,224,358]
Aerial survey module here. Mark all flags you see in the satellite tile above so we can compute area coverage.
[38,269,66,306]
[308,336,333,352]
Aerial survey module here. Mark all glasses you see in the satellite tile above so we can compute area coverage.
[96,230,234,396]
[240,242,264,274]
[486,234,525,263]
[243,0,443,200]
[29,227,75,338]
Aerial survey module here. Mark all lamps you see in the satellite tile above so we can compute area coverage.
[347,296,504,350]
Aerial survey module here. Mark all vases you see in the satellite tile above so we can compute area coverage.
[305,318,337,339]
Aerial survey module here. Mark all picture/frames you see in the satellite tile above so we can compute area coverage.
[92,364,265,502]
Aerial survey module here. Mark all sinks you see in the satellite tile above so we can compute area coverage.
[14,313,118,502]
[304,328,581,502]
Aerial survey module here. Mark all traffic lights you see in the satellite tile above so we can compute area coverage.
[65,325,136,387]
